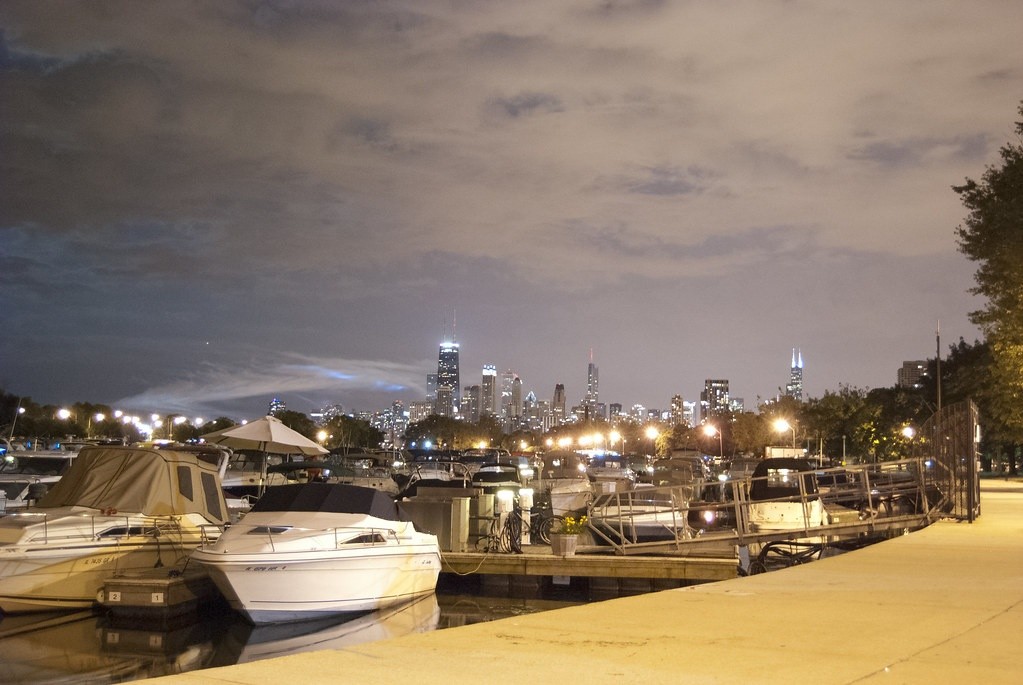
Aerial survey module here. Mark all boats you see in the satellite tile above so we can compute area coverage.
[189,480,442,627]
[0,447,829,613]
[211,592,440,664]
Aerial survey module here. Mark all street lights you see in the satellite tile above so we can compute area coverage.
[842,435,846,463]
[775,420,796,448]
[608,432,625,455]
[702,425,723,459]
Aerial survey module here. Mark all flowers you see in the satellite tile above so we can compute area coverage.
[550,516,589,534]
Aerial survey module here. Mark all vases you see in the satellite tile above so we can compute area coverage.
[549,533,578,556]
